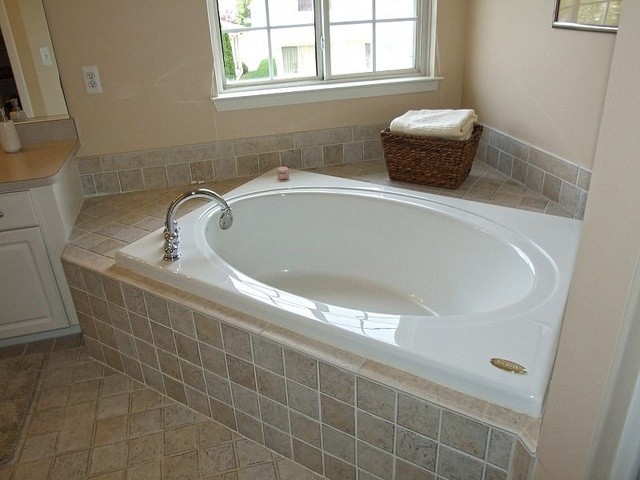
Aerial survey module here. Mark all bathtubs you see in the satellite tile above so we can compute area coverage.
[114,165,584,420]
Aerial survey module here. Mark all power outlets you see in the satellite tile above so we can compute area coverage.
[83,65,101,94]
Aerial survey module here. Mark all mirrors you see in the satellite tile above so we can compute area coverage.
[0,27,27,121]
[552,0,621,35]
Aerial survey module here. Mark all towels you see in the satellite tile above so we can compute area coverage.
[390,106,477,143]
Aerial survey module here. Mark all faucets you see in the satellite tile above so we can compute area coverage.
[164,187,234,261]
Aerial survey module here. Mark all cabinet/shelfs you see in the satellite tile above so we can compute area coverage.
[0,157,83,346]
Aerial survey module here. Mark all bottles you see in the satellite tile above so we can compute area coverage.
[5,97,25,121]
[0,105,22,153]
